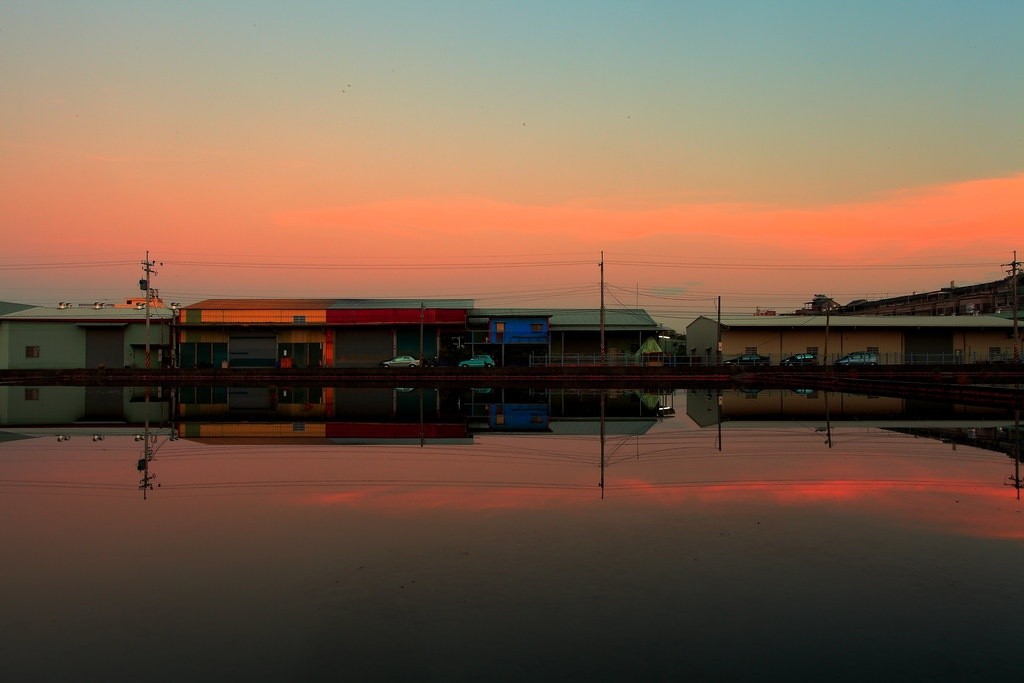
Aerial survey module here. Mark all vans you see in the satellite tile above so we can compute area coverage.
[838,352,878,367]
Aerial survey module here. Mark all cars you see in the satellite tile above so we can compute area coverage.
[781,353,818,367]
[458,355,495,368]
[381,356,421,368]
[724,352,771,366]
[396,387,415,392]
[732,382,770,394]
[795,388,818,394]
[471,388,492,394]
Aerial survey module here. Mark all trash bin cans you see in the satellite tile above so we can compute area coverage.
[280,358,295,368]
